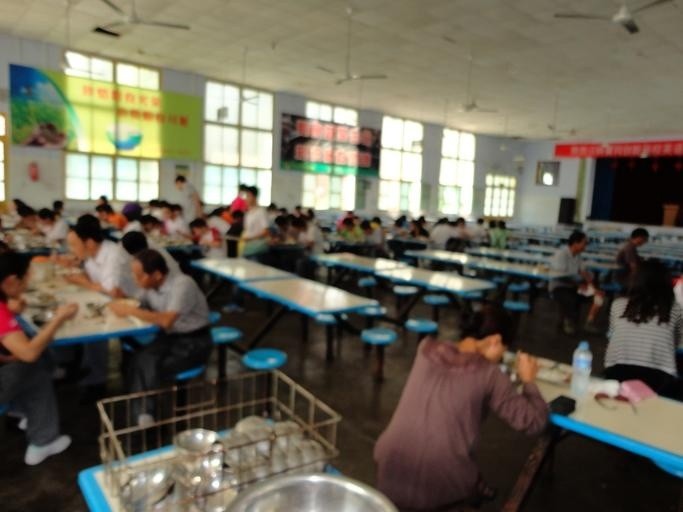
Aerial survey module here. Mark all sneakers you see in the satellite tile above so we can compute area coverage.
[24,435,72,465]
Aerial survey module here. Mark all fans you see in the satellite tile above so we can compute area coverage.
[92,0,190,34]
[553,0,675,35]
[312,7,386,86]
[457,54,499,113]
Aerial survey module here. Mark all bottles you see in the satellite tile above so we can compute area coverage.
[572,342,594,398]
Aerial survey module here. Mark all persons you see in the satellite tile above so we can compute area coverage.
[373,304,548,512]
[337,211,510,258]
[616,228,649,289]
[0,174,331,465]
[548,232,594,338]
[21,123,65,147]
[604,260,683,399]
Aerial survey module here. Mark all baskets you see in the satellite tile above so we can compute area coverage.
[96,368,342,512]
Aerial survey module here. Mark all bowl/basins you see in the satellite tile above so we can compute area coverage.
[31,312,62,332]
[108,299,140,318]
[103,130,143,150]
[120,414,324,511]
[220,472,393,511]
[498,349,514,373]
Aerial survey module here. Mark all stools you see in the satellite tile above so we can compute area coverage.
[124,334,157,353]
[4,226,683,321]
[175,369,208,389]
[210,328,243,381]
[358,305,388,353]
[362,329,398,384]
[406,318,440,347]
[503,302,531,346]
[243,349,289,416]
